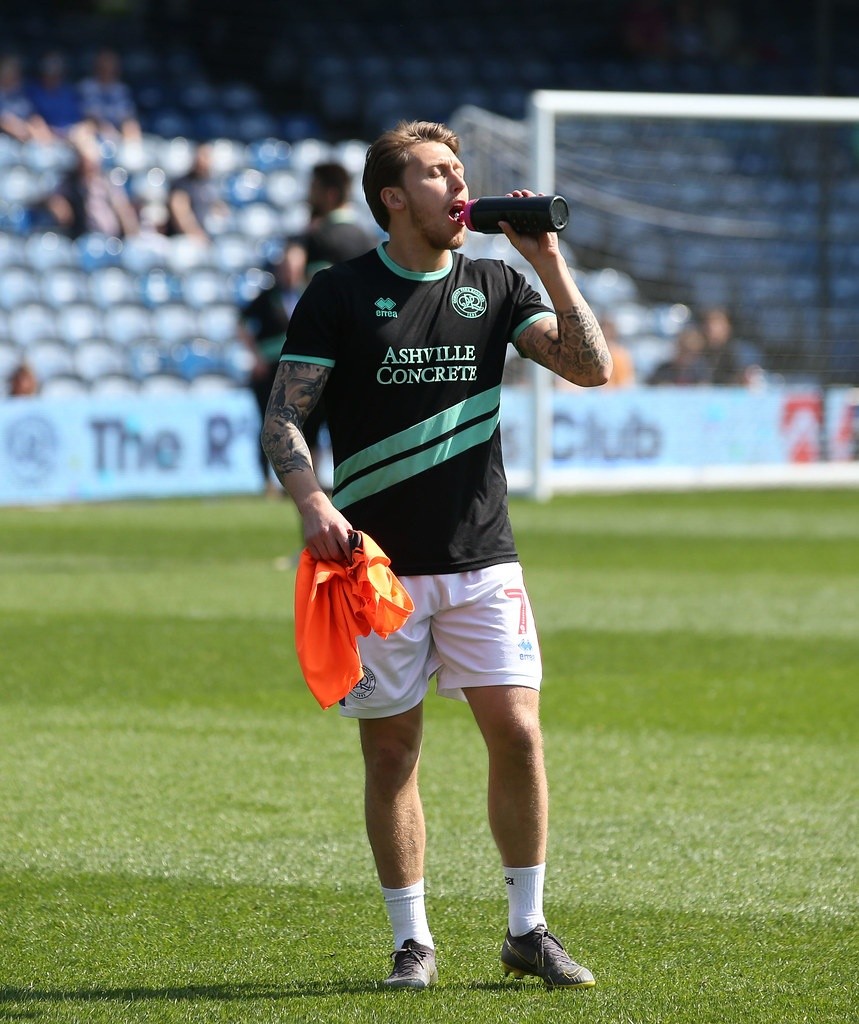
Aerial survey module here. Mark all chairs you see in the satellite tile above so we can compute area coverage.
[0,110,762,406]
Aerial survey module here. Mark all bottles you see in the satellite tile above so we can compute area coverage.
[456,195,569,234]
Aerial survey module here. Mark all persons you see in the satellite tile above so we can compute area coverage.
[646,305,748,382]
[286,161,380,292]
[240,258,322,495]
[260,119,612,989]
[1,46,232,241]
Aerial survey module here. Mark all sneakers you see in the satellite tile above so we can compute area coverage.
[383,938,439,993]
[501,924,596,989]
